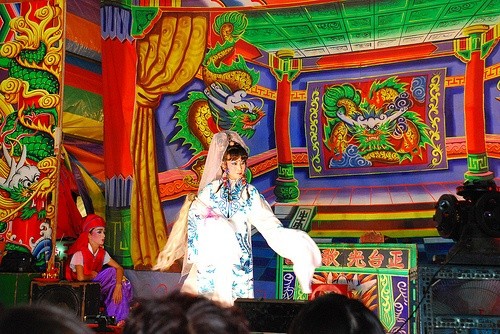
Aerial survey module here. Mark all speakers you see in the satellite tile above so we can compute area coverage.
[30,281,100,325]
[234,297,309,334]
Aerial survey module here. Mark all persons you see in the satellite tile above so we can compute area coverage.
[67,213,132,325]
[0,301,98,334]
[121,290,253,334]
[181,130,323,309]
[289,291,386,334]
